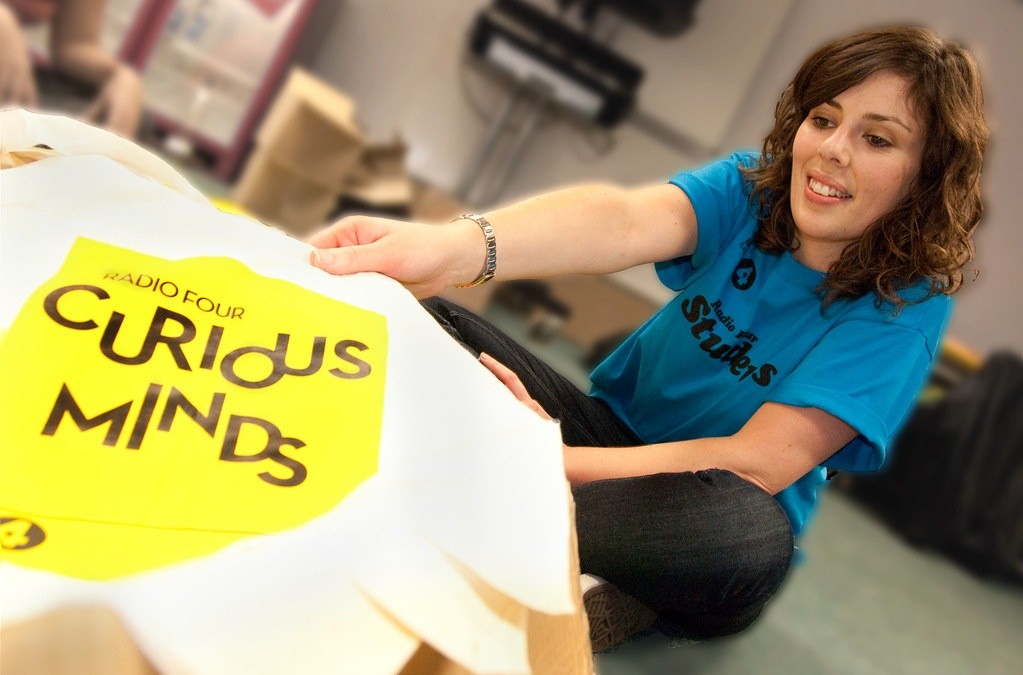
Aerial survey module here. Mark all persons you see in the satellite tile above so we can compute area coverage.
[309,22,993,652]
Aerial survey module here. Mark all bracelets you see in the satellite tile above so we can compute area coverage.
[453,214,495,289]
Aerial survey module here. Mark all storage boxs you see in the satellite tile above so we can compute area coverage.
[237,69,412,240]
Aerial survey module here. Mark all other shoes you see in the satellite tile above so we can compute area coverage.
[580,573,659,652]
